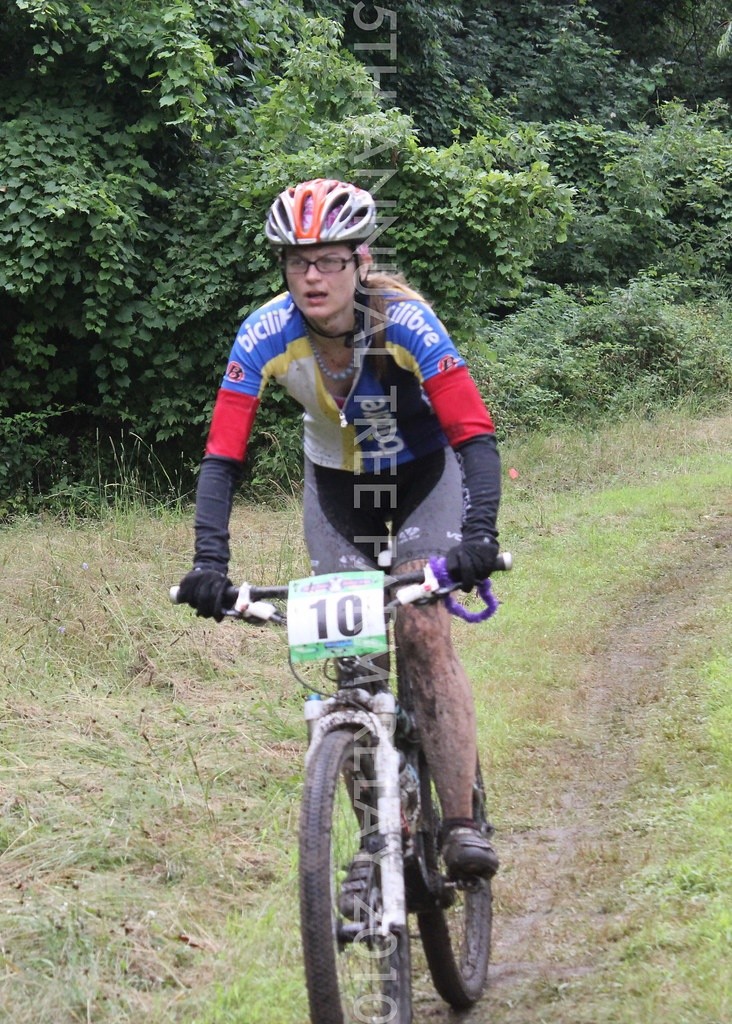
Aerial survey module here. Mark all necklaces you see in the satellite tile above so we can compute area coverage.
[308,340,355,380]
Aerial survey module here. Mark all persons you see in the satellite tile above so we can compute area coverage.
[175,179,502,946]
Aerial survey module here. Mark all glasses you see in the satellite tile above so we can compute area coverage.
[281,255,354,274]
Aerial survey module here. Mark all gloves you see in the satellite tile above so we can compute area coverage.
[446,538,499,593]
[178,568,233,623]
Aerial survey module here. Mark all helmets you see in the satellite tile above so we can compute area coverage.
[264,178,376,246]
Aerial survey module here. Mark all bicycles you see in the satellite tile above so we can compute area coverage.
[167,547,509,1024]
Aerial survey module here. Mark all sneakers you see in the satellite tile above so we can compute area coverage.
[335,858,379,922]
[443,828,499,882]
